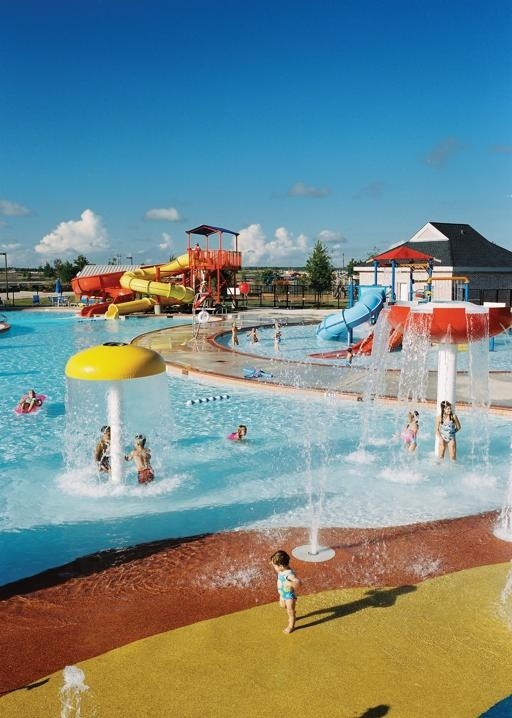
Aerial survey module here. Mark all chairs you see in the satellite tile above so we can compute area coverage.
[33,295,39,304]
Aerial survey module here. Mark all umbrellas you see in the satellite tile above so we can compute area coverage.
[55,278,63,307]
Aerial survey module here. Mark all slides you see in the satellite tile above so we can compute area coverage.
[315,288,386,339]
[72,254,195,319]
[309,325,403,359]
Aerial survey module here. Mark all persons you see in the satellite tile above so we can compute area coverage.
[435,399,461,461]
[193,242,200,250]
[270,549,301,633]
[92,424,112,473]
[401,408,420,452]
[226,424,247,441]
[124,433,155,484]
[272,323,282,350]
[345,347,353,367]
[231,321,240,345]
[385,286,397,303]
[247,327,258,342]
[18,388,42,413]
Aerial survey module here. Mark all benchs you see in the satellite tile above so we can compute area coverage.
[48,296,72,307]
